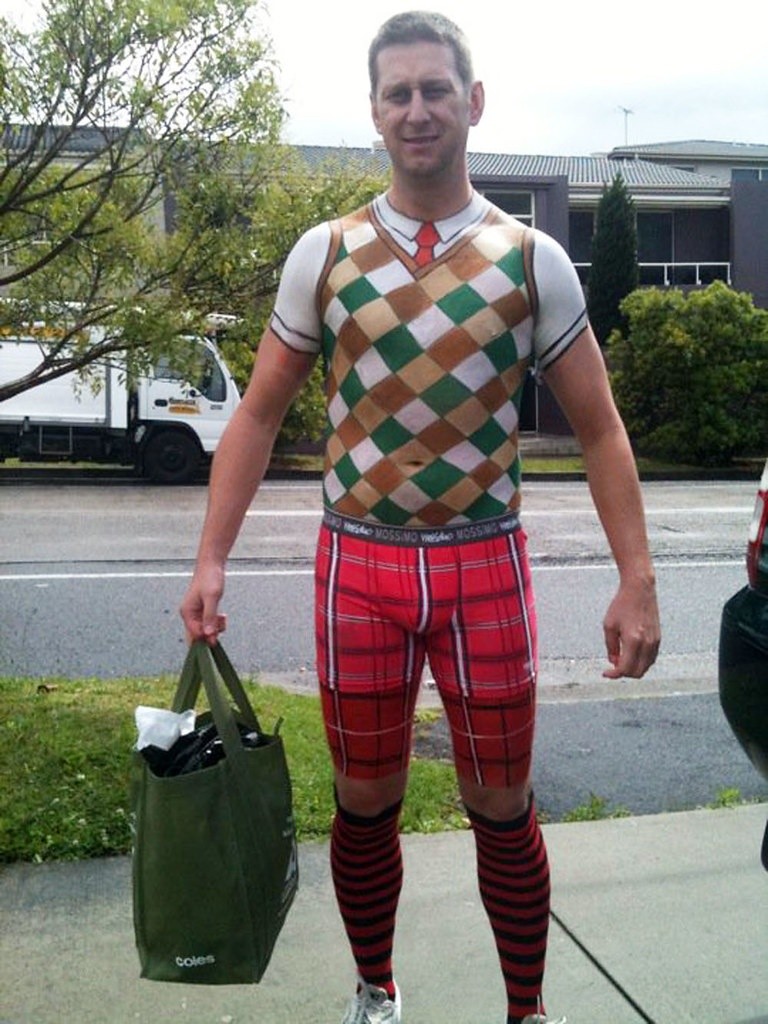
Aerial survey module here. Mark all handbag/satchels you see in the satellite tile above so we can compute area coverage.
[134,636,299,983]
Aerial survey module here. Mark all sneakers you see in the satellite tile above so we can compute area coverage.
[343,977,403,1022]
[508,994,565,1024]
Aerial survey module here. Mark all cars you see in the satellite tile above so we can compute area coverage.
[713,456,768,783]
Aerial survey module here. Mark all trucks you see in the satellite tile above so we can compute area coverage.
[1,298,245,485]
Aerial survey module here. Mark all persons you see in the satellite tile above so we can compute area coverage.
[179,13,661,1023]
